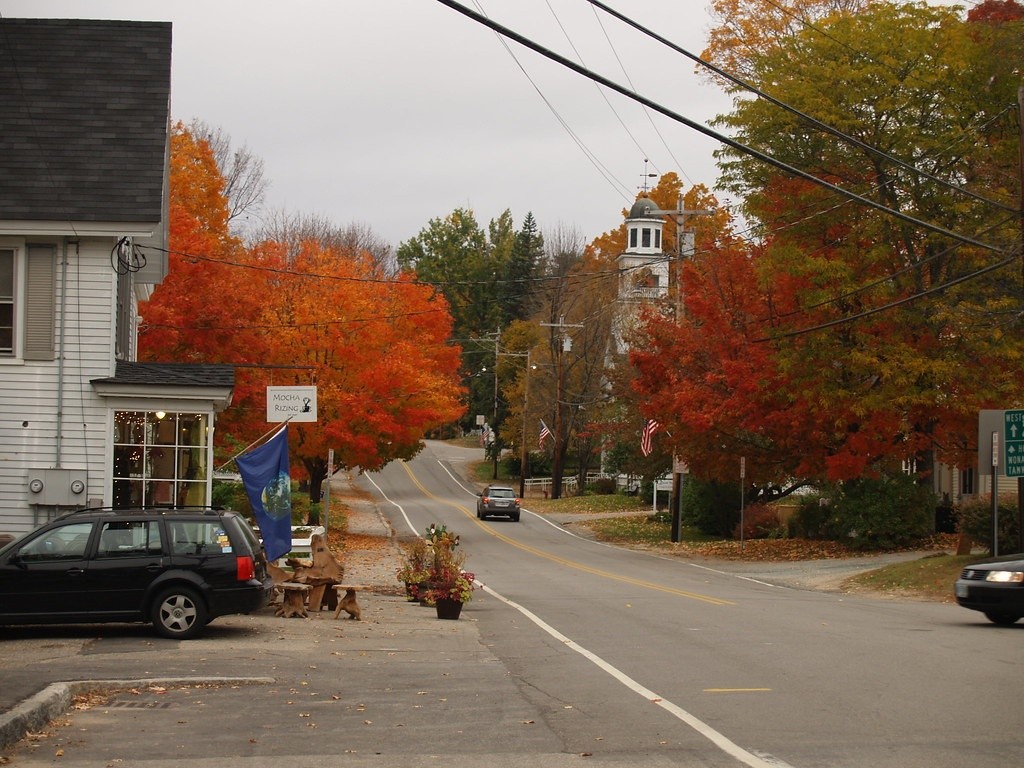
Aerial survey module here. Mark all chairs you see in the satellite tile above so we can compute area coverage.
[504,491,510,497]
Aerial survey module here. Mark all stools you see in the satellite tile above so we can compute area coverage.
[273,582,315,619]
[330,585,373,622]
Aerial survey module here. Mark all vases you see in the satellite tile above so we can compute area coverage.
[406,582,466,621]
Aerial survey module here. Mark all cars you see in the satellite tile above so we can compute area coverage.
[475,485,521,522]
[952,551,1024,625]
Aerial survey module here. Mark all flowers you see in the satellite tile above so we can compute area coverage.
[393,521,486,606]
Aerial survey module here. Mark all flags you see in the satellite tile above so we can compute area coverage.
[234,424,292,562]
[481,428,489,438]
[539,423,549,449]
[640,417,660,458]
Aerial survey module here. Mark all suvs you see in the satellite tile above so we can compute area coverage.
[0,502,279,639]
